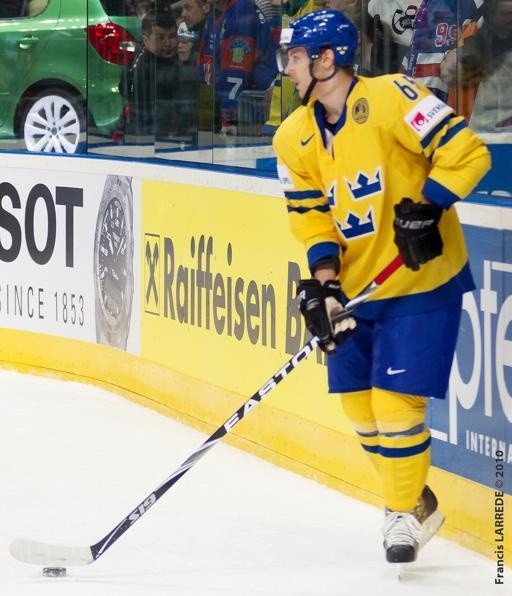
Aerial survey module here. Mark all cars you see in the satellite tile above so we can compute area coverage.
[0,0,145,154]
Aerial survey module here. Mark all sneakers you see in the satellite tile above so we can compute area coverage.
[412,485,437,524]
[384,507,418,562]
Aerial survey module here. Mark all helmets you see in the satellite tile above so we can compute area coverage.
[281,10,358,67]
[178,22,199,38]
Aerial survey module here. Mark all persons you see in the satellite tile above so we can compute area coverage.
[118,0,512,148]
[271,8,492,563]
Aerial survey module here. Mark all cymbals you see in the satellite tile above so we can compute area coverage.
[7,255,406,565]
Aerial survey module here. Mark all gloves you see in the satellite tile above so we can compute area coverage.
[393,197,443,272]
[297,279,359,354]
